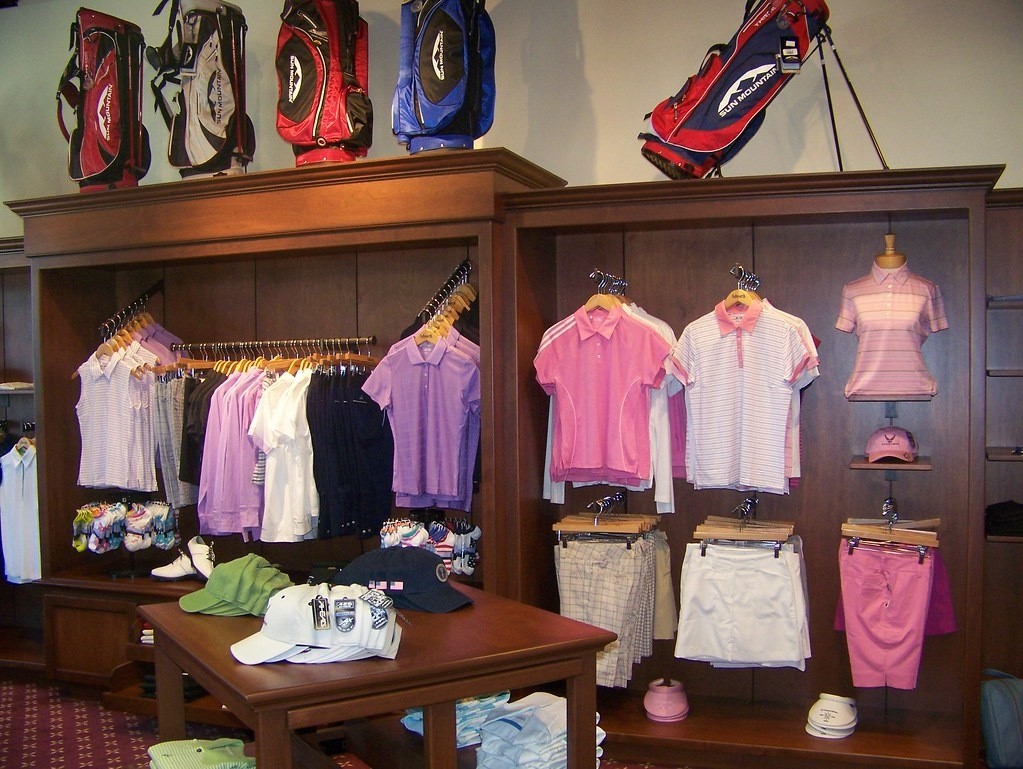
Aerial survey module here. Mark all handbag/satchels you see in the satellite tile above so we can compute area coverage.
[978,668,1023,769]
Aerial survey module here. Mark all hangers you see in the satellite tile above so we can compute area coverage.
[692,496,798,560]
[14,422,37,452]
[415,259,478,347]
[724,263,764,313]
[840,500,942,565]
[66,294,382,380]
[551,493,664,551]
[584,270,634,316]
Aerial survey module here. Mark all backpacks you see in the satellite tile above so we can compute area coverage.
[146,0,256,181]
[55,7,152,195]
[391,0,496,155]
[275,0,373,168]
[637,0,830,180]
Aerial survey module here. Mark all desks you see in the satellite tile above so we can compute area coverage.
[135,581,617,769]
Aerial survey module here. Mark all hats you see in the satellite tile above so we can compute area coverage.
[328,546,474,613]
[804,692,858,739]
[864,426,919,463]
[643,678,690,724]
[178,552,295,618]
[230,582,403,665]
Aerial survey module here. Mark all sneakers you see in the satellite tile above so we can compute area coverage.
[186,535,215,581]
[150,549,198,582]
